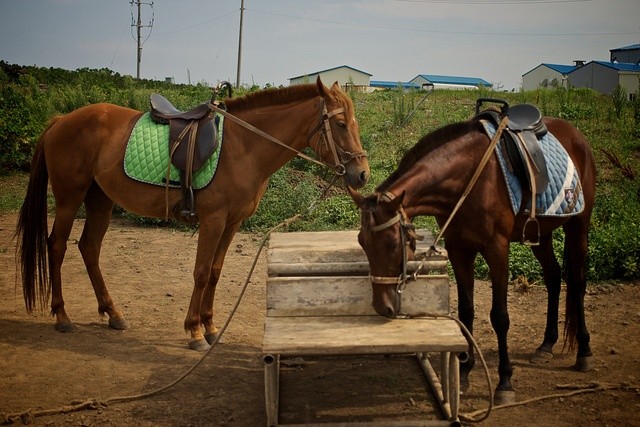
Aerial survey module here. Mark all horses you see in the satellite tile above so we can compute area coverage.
[4,73,371,351]
[344,114,597,407]
